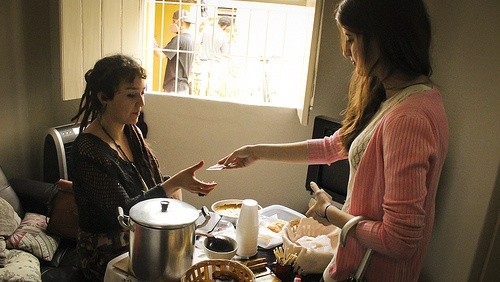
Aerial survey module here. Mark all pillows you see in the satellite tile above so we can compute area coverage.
[0,197,60,282]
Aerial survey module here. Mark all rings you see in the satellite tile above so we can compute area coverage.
[313,189,322,195]
[199,187,204,192]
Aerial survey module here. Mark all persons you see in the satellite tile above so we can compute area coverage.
[152,9,197,95]
[69,54,217,282]
[222,0,450,282]
[197,16,237,96]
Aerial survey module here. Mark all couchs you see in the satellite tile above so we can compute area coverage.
[0,166,83,282]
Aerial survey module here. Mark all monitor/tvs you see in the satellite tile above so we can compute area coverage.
[305,115,350,204]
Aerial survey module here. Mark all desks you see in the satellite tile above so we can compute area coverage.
[104,204,323,282]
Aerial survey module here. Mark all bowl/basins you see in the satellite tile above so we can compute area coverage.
[201,235,238,259]
[212,199,263,220]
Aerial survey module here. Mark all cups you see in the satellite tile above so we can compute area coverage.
[236,197,260,259]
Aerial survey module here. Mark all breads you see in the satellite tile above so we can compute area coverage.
[266,220,285,233]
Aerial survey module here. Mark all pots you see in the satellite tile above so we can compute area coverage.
[117,197,211,282]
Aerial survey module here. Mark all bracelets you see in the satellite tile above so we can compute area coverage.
[314,204,332,225]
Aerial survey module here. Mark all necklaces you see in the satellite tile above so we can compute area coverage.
[96,116,157,192]
